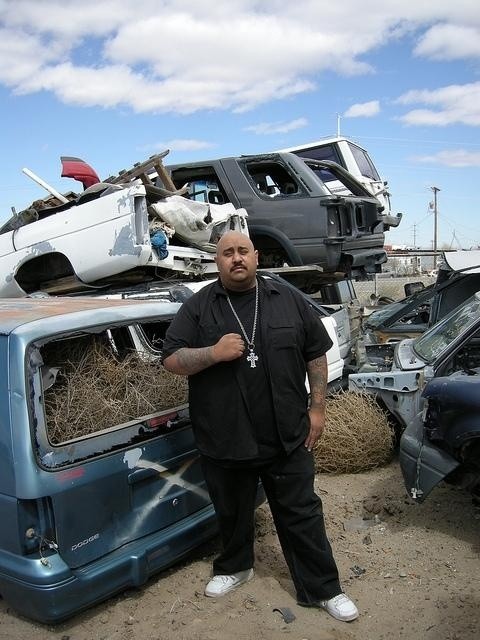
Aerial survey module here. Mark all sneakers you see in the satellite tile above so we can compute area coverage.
[315,593,359,622]
[203,567,255,598]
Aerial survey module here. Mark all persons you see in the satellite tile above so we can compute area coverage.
[160,227,359,623]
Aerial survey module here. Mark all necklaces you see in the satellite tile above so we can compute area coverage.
[222,276,260,370]
